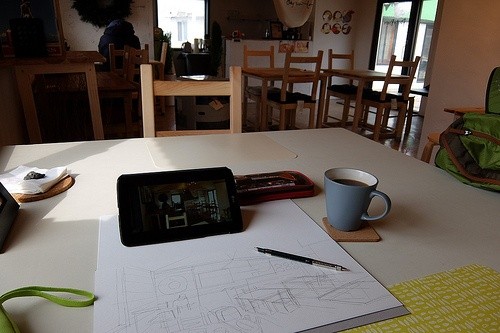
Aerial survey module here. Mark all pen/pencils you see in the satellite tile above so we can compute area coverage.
[255,247,349,272]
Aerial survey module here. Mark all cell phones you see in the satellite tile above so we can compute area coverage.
[117,167,244,247]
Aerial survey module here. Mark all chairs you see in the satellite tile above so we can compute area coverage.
[241,45,290,129]
[421,66,500,195]
[267,46,324,129]
[325,49,372,127]
[75,43,169,137]
[139,64,242,139]
[359,55,420,141]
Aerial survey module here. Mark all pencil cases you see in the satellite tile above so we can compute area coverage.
[234,171,315,206]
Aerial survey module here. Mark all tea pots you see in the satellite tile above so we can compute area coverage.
[181,41,191,53]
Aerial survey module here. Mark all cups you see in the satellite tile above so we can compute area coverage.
[324,167,391,232]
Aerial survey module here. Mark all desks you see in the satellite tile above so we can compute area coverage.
[15,50,105,144]
[244,66,328,133]
[0,127,500,333]
[319,67,416,131]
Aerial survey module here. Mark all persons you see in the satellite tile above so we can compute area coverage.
[162,200,169,209]
[98,13,140,70]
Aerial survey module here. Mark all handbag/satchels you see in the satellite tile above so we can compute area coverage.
[433,66,500,192]
